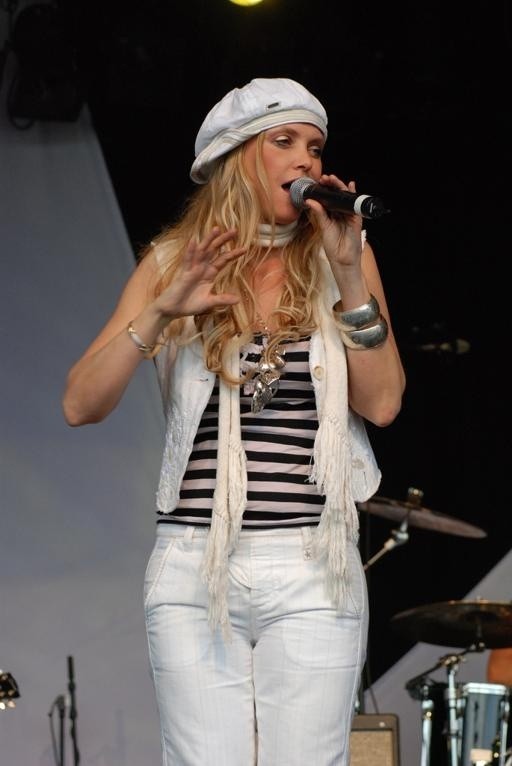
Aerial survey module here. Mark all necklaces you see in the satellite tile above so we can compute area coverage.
[244,292,287,414]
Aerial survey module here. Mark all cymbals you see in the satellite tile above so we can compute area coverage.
[393,603,510,651]
[361,502,486,539]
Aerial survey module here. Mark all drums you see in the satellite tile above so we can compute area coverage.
[423,682,510,765]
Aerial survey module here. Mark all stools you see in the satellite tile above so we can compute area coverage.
[347,713,401,766]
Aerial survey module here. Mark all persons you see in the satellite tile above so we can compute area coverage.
[62,77,409,766]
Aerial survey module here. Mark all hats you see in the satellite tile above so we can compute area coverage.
[190,77,329,185]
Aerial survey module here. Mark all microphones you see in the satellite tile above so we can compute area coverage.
[290,178,383,220]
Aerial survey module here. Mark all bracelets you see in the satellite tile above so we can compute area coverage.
[332,292,379,332]
[338,313,388,351]
[126,320,155,352]
